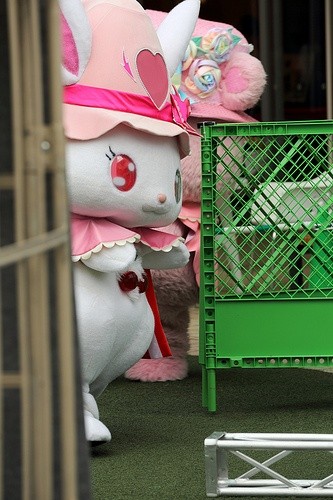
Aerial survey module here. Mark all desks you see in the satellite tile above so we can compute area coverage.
[212,176,248,294]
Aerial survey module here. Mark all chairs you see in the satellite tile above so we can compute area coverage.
[251,166,333,292]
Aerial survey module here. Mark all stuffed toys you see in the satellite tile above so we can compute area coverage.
[123,5,266,380]
[59,0,205,444]
